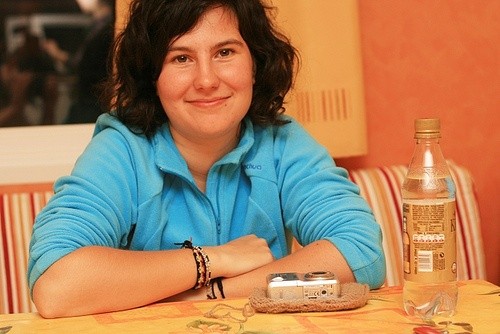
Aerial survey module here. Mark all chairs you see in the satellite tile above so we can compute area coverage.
[0,182,57,314]
[291,154,487,288]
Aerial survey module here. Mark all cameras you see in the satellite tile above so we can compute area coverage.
[266,269,342,301]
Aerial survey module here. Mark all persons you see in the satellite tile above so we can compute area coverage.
[21,0,387,319]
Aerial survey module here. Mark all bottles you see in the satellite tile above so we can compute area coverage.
[401,118,457,320]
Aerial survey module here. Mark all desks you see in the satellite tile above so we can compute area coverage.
[0,279,500,334]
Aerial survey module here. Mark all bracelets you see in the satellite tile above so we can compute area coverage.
[207,278,225,298]
[175,240,211,289]
